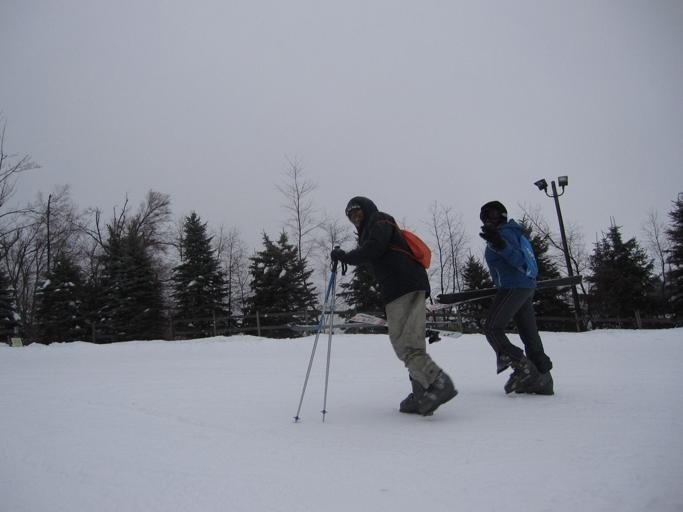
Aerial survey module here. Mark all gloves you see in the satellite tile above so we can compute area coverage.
[331,248,352,264]
[480,223,502,246]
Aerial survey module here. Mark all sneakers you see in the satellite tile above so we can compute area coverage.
[399,370,457,416]
[505,352,554,395]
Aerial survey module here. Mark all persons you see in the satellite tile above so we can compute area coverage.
[478,201,555,397]
[331,195,457,416]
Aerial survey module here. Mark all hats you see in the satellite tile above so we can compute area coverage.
[345,200,361,215]
[480,201,507,218]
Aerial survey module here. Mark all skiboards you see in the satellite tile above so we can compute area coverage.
[426,275,582,310]
[286,312,462,343]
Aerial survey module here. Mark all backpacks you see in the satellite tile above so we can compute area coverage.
[510,228,539,279]
[375,220,431,268]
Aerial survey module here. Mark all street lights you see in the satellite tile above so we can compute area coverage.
[535,176,586,330]
